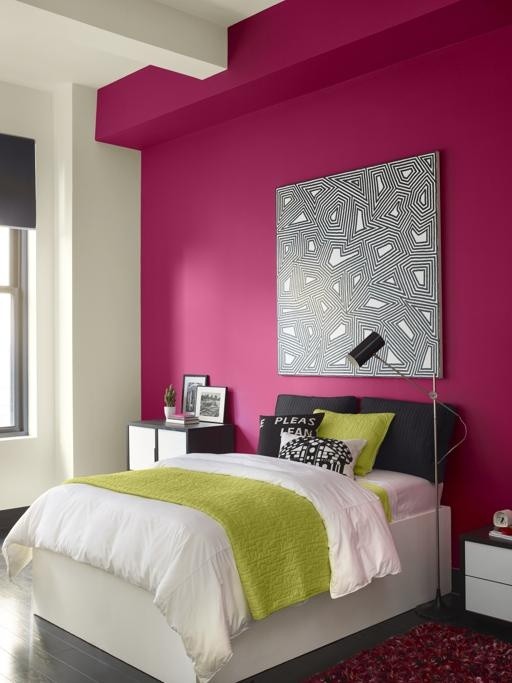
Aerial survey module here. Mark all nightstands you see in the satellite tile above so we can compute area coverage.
[458,523,512,641]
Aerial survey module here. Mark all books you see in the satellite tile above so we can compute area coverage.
[166,413,199,426]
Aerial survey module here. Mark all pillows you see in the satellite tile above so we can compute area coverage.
[252,407,398,481]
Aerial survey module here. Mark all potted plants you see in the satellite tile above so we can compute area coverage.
[163,384,178,420]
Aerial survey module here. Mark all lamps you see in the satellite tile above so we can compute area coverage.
[345,332,471,625]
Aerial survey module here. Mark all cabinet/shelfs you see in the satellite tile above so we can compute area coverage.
[127,416,235,472]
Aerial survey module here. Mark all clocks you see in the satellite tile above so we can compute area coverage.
[493,509,512,530]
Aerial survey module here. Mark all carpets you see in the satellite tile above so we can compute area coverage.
[297,619,512,683]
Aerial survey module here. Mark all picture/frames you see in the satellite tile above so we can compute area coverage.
[181,373,229,424]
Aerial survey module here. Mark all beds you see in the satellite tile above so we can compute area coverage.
[1,392,463,683]
